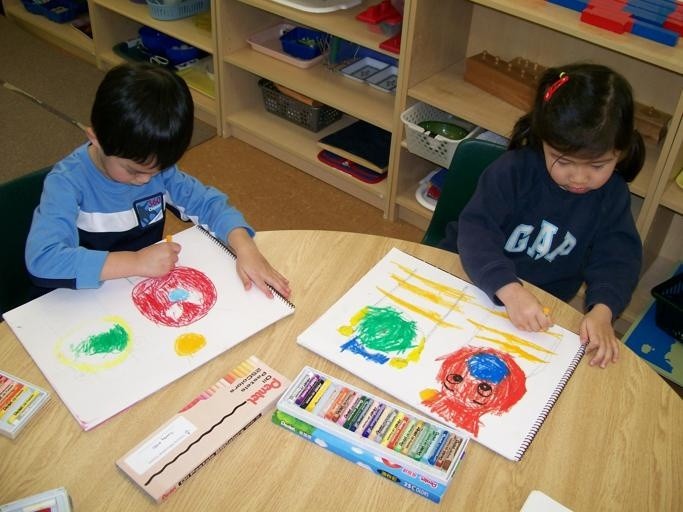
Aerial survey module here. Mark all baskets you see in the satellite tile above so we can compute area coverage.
[650,270,682,345]
[146,0,210,21]
[399,101,486,170]
[257,78,341,133]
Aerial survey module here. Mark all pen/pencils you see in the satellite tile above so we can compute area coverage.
[295,370,464,475]
[1,376,45,425]
[542,308,550,324]
[166,236,175,245]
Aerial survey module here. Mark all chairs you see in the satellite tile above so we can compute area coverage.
[420,141,508,256]
[0,166,51,323]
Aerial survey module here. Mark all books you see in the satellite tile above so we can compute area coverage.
[70,17,93,40]
[295,246,585,463]
[314,120,390,175]
[1,223,295,431]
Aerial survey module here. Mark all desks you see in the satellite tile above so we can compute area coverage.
[0,230,681,510]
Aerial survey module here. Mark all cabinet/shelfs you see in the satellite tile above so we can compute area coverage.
[218,1,407,223]
[387,2,682,310]
[97,0,215,136]
[3,2,93,59]
[611,115,683,397]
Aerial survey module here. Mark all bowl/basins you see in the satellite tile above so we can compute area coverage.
[418,121,468,140]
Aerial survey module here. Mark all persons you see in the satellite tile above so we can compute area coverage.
[436,62,642,369]
[24,61,291,302]
